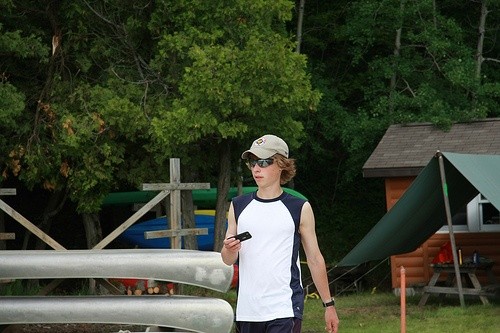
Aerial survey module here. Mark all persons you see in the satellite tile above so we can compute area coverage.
[221,135,340,333]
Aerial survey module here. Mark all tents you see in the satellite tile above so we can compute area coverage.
[335,150,500,268]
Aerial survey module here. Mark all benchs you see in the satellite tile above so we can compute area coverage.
[409,281,448,288]
[480,284,499,293]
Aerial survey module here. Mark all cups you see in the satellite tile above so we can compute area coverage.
[458,249,463,264]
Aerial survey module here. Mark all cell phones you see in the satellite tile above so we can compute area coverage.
[227,231,252,242]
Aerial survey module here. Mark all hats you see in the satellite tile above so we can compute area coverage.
[241,135,289,159]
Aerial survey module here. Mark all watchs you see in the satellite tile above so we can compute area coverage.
[323,297,335,307]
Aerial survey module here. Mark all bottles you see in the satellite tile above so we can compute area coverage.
[472,251,479,265]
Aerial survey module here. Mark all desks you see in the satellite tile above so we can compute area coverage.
[418,262,490,307]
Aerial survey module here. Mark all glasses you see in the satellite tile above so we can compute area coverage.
[248,158,276,168]
